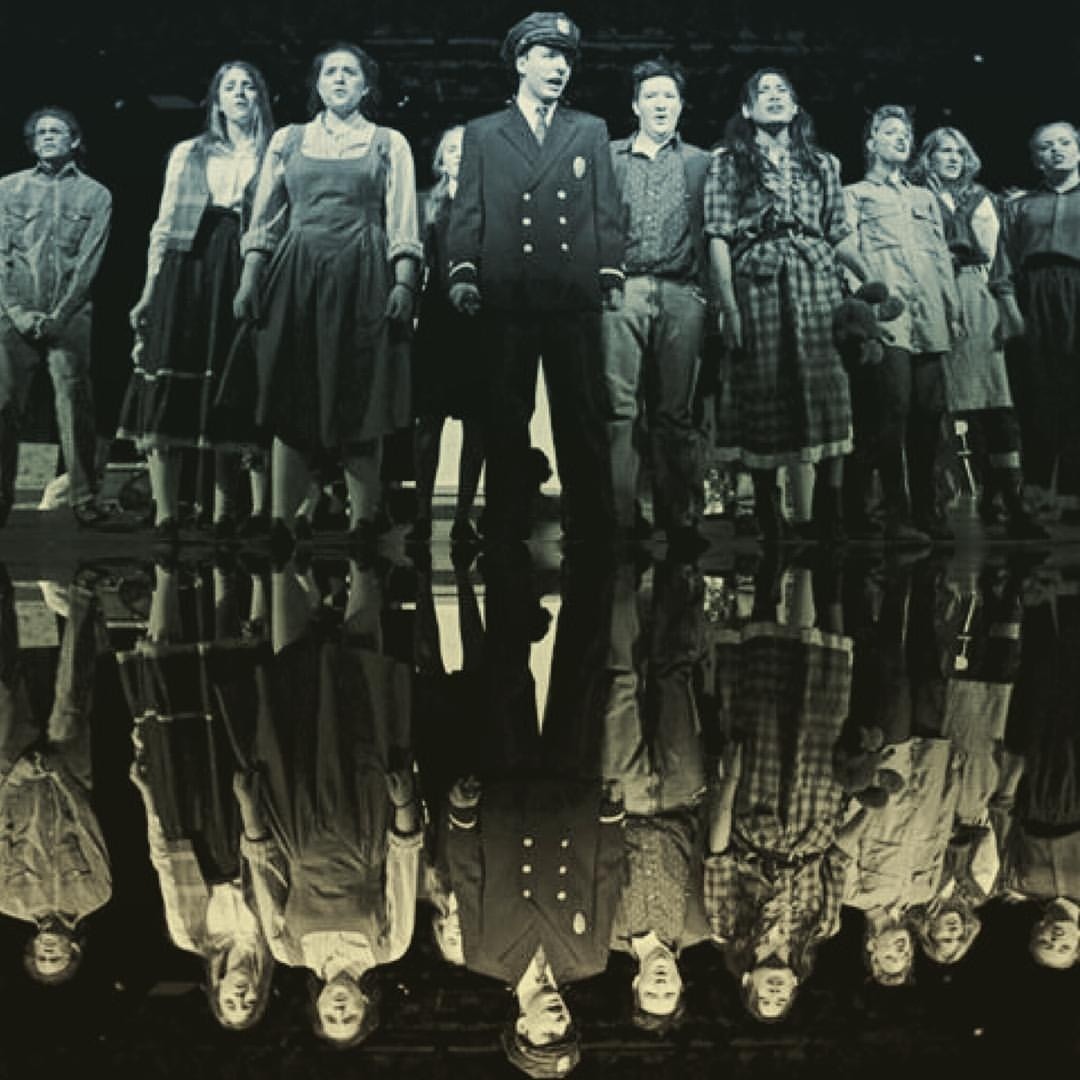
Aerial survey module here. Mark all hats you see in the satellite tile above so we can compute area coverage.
[501,11,581,63]
[501,1029,581,1080]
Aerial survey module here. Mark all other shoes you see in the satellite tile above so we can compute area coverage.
[69,545,1054,574]
[74,501,1080,545]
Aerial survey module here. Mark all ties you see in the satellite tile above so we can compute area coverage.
[535,106,548,149]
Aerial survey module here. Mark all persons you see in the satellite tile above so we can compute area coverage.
[0,565,1080,1075]
[3,9,1080,563]
